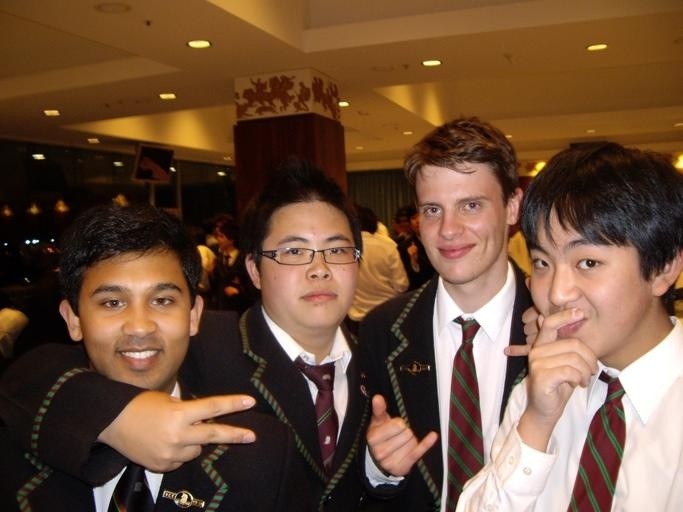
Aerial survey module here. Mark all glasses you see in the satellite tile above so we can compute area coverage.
[253,244,363,267]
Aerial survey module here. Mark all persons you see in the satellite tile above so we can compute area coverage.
[0,201,324,510]
[323,113,535,511]
[452,137,681,512]
[0,160,365,510]
[1,197,683,376]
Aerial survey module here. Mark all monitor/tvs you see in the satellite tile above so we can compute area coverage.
[130,143,175,184]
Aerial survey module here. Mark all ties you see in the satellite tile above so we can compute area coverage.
[223,254,231,266]
[107,463,157,511]
[566,370,627,511]
[293,355,340,483]
[443,316,487,511]
